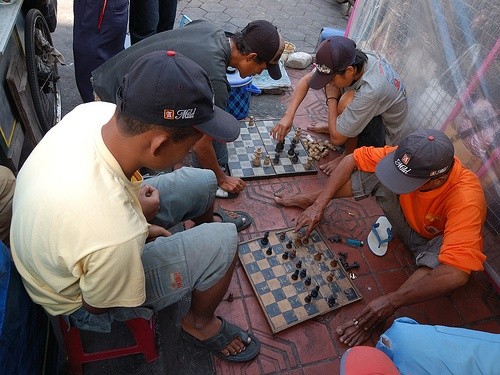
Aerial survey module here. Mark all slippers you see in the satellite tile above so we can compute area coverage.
[213,207,252,232]
[367,216,393,256]
[181,316,261,363]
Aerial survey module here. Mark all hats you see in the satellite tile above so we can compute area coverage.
[308,36,356,89]
[116,51,241,143]
[375,130,454,194]
[241,20,282,80]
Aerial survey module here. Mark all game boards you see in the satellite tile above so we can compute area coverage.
[225,117,318,179]
[237,223,364,335]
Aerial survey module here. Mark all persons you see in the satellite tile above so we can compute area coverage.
[0,0,285,246]
[270,36,408,174]
[273,128,500,375]
[9,50,262,363]
